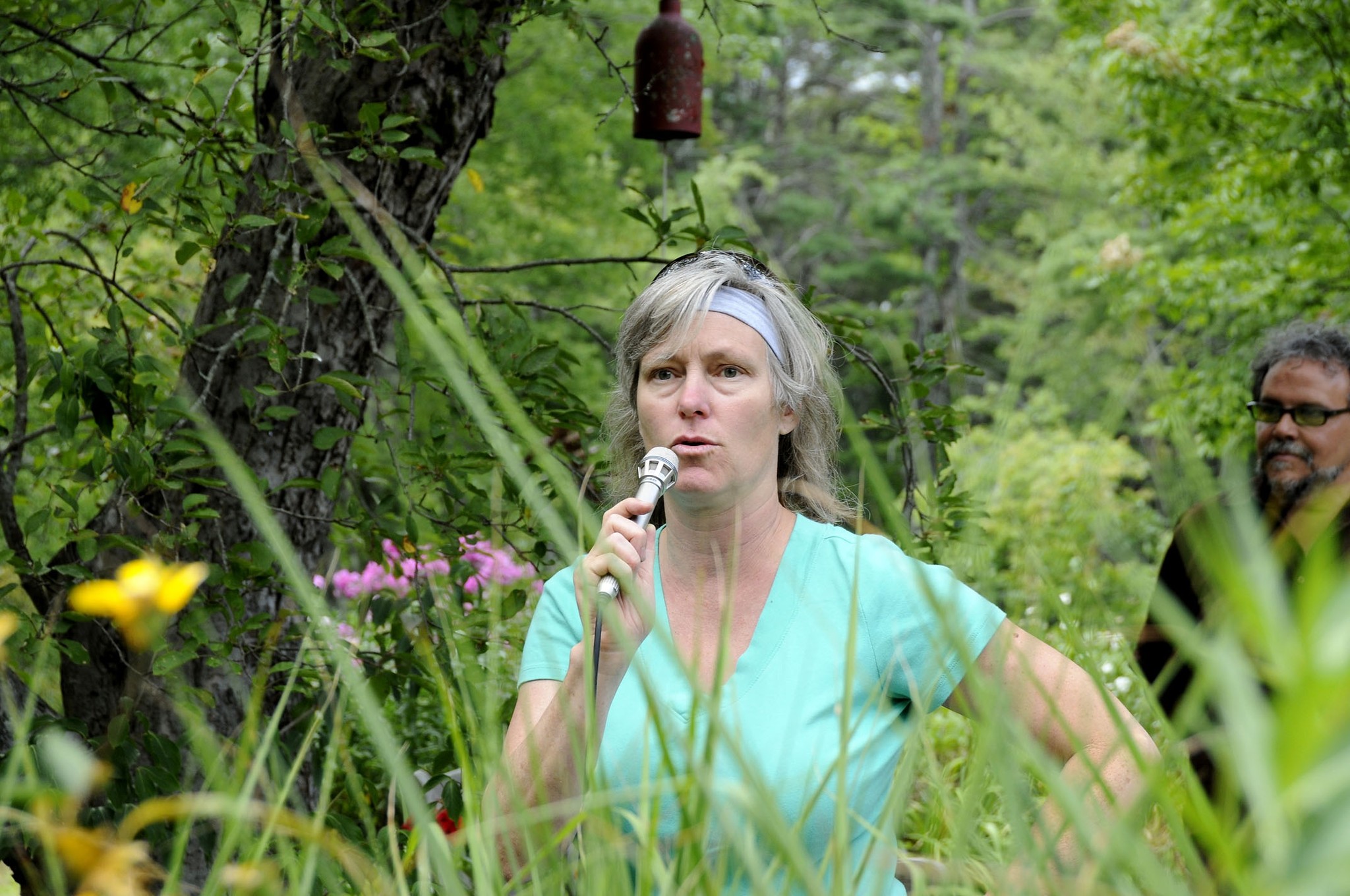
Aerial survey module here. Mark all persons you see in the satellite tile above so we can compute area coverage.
[480,251,1161,896]
[1136,322,1350,884]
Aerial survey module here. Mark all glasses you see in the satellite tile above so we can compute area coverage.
[1247,397,1350,428]
[648,249,785,294]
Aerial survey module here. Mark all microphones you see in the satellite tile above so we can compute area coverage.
[595,447,680,606]
